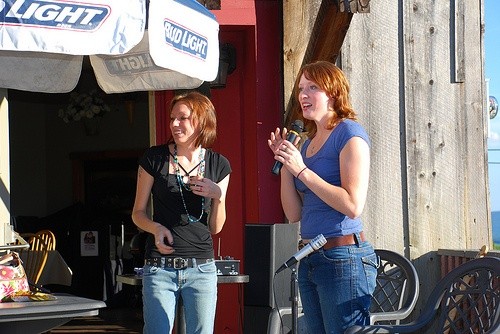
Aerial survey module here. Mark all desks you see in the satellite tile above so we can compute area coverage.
[116,274,250,334]
[0,293,107,334]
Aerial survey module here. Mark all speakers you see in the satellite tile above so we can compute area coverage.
[244,221,303,308]
[242,307,304,334]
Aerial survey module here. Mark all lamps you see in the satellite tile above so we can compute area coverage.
[206,43,235,88]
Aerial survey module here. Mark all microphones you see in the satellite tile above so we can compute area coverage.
[272,119,304,174]
[275,234,328,274]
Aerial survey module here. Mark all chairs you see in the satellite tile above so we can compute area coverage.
[267,249,500,334]
[11,229,58,291]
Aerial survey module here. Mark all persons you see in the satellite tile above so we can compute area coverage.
[268,61,379,334]
[131,87,232,334]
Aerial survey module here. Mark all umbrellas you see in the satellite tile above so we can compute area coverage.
[0,0,219,94]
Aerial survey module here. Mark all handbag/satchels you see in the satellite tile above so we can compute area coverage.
[0,252,32,302]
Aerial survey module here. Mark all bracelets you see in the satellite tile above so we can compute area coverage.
[297,167,307,178]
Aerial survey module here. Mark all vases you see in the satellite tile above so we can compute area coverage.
[82,117,100,136]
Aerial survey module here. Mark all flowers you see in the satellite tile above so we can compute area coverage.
[57,88,113,123]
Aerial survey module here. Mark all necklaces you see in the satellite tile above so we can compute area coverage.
[173,143,205,222]
[311,126,328,152]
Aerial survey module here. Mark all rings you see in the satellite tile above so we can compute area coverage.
[200,187,202,192]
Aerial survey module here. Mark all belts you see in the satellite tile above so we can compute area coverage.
[145,257,213,269]
[298,231,365,252]
